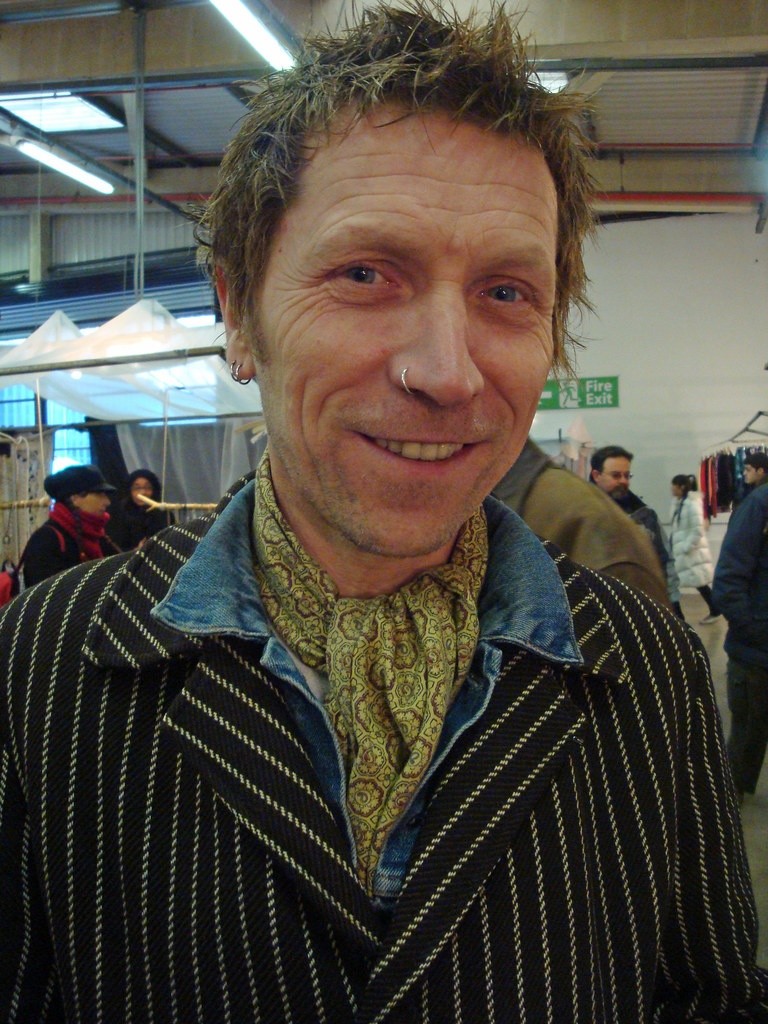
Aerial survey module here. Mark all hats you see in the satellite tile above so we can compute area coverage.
[43,466,117,502]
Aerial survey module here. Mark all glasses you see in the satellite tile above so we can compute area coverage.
[596,471,634,480]
[131,481,154,493]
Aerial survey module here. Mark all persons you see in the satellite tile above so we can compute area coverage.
[4,3,768,1023]
[588,446,687,622]
[667,452,768,810]
[22,466,176,589]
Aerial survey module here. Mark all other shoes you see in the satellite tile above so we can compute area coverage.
[700,614,724,625]
